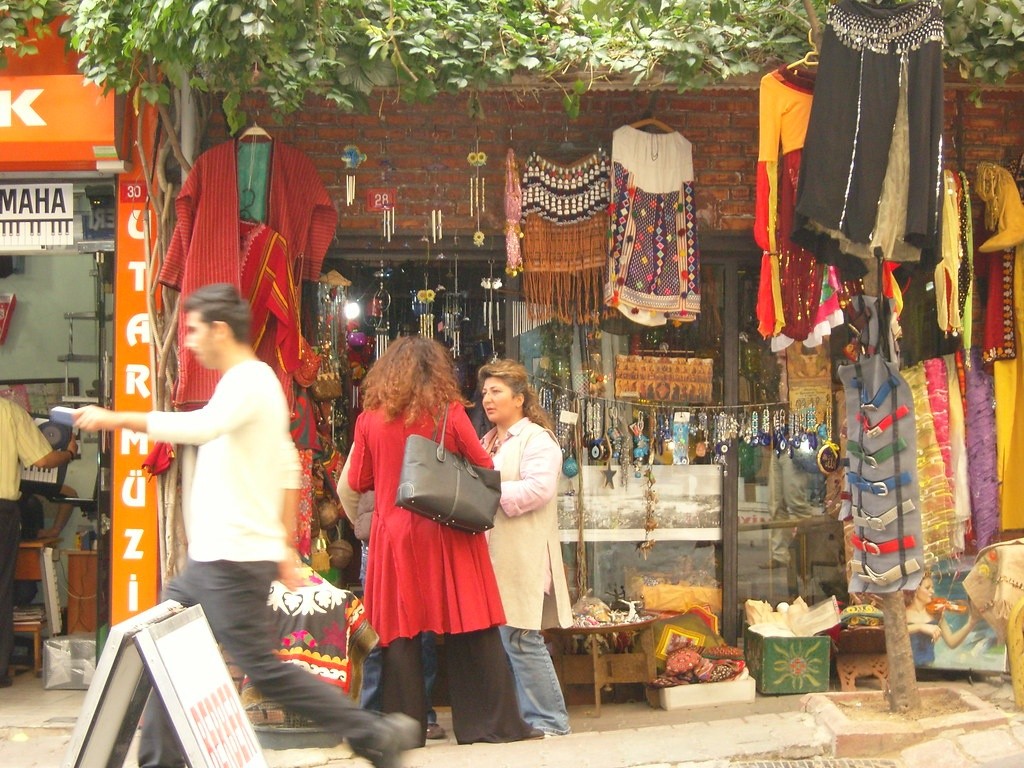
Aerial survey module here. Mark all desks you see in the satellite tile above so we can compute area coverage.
[9,536,99,674]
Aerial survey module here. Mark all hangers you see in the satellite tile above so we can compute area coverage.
[786,28,820,70]
[238,111,272,143]
[240,189,256,217]
[531,122,602,158]
[630,108,675,133]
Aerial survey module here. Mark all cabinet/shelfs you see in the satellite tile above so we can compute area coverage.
[57,311,113,443]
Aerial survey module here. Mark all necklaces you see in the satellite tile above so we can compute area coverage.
[525,378,818,491]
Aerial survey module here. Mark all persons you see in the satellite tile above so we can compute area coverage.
[73,284,421,768]
[0,394,79,687]
[475,358,573,736]
[337,334,544,745]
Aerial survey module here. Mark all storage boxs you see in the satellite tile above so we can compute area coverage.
[659,675,756,711]
[43,636,96,690]
[743,628,830,694]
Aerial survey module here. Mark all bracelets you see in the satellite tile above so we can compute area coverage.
[66,449,74,462]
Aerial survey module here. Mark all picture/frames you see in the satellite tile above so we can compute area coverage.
[0,377,79,440]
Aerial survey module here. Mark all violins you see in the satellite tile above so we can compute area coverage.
[925,595,970,614]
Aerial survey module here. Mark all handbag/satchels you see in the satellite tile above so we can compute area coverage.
[393,402,501,533]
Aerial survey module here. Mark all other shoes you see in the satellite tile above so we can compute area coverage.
[375,713,420,768]
[426,723,445,741]
[523,729,545,742]
[0,677,12,689]
